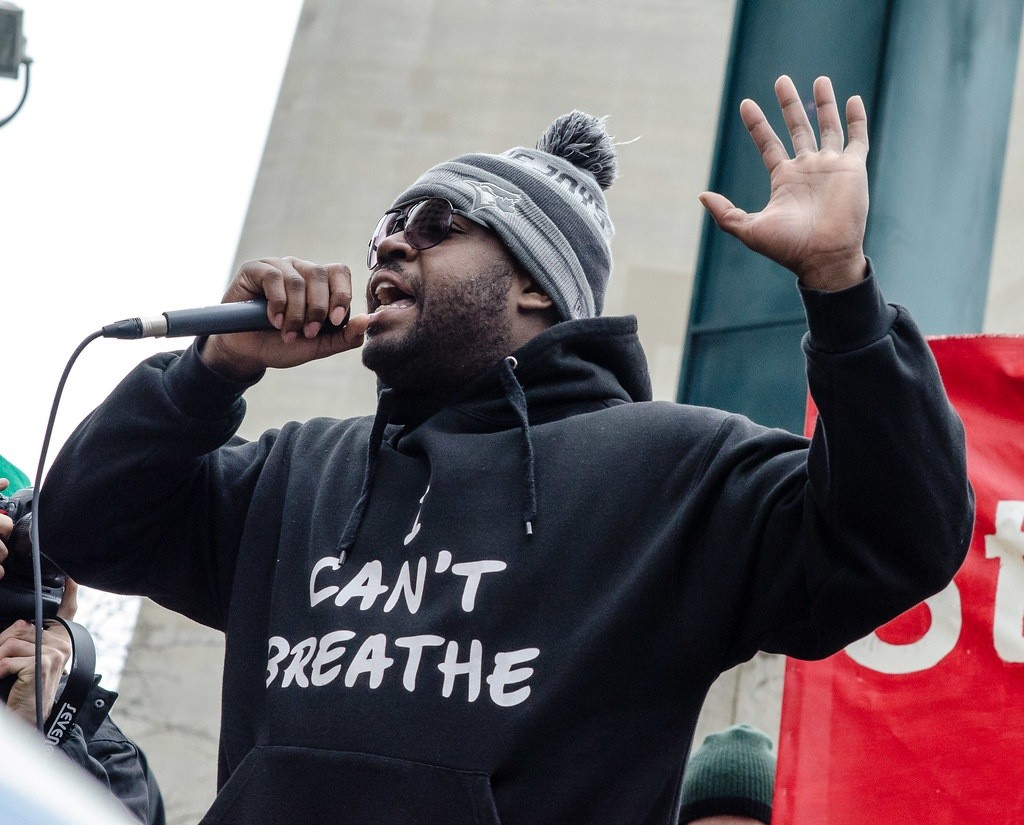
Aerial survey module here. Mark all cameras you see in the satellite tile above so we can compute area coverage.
[0,488,65,630]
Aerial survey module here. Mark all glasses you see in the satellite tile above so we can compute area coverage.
[365,196,491,270]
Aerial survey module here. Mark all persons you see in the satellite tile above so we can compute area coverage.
[0,453,165,825]
[673,721,790,825]
[39,74,976,825]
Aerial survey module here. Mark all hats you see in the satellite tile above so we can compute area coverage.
[390,111,618,322]
[677,723,778,825]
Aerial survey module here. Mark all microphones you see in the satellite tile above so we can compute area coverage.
[101,296,351,341]
